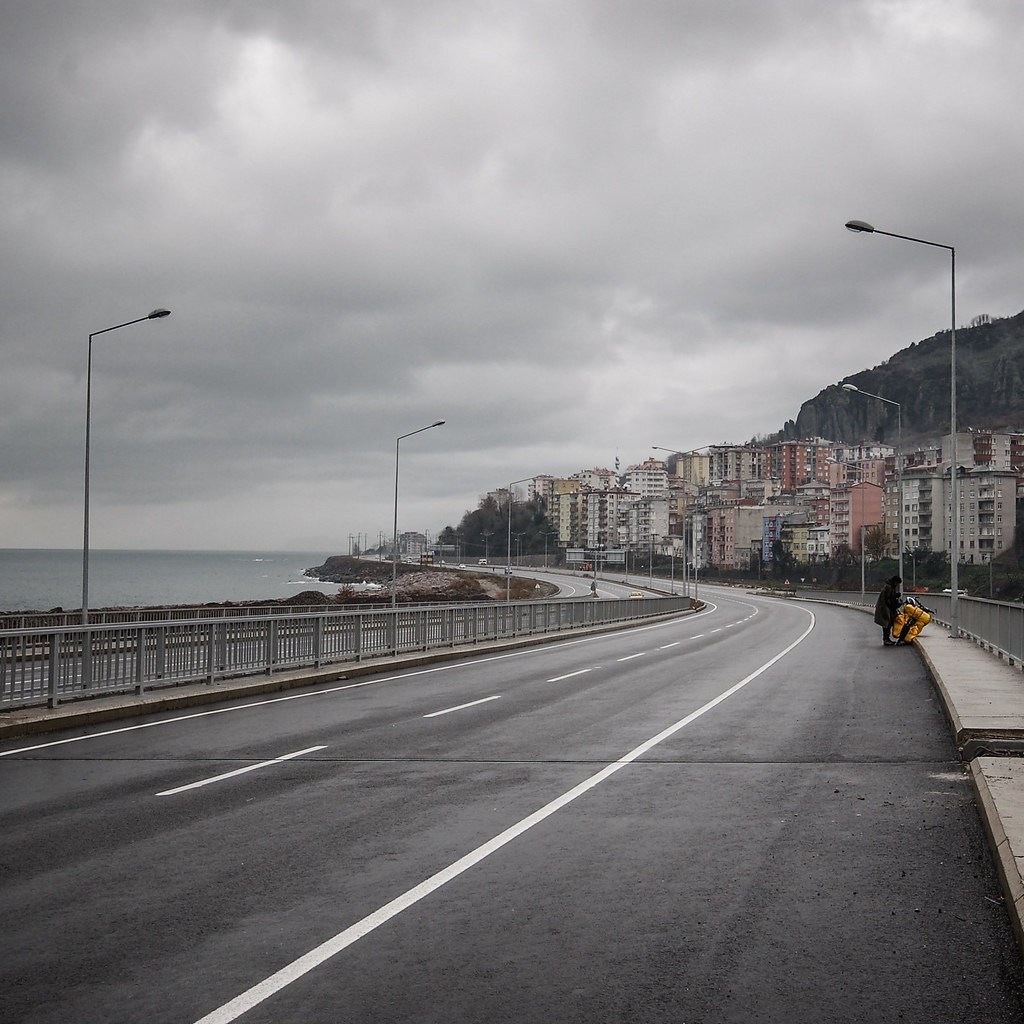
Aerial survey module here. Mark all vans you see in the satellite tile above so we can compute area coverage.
[478,558,487,565]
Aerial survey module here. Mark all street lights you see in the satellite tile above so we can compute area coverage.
[505,473,548,614]
[652,444,717,595]
[842,384,905,600]
[390,419,446,611]
[81,307,171,626]
[844,219,958,645]
[826,455,865,605]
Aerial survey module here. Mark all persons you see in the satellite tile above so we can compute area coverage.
[875,576,904,645]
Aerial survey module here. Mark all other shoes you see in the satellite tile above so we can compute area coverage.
[884,638,896,645]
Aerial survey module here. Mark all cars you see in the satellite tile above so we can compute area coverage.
[406,559,412,563]
[458,564,466,569]
[628,592,644,599]
[505,568,512,574]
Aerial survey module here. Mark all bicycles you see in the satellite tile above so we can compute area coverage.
[893,597,937,645]
